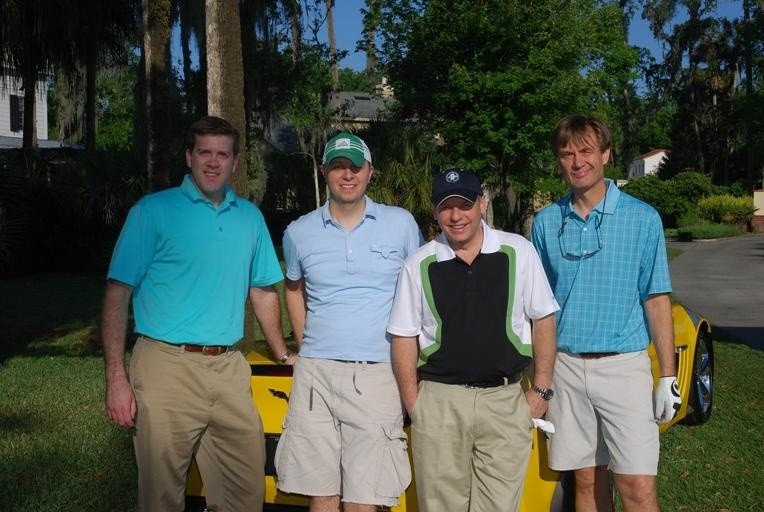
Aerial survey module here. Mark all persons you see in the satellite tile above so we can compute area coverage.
[272,132,429,512]
[530,115,681,511]
[100,116,298,511]
[385,168,562,512]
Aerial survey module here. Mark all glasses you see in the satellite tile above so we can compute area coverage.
[559,215,602,259]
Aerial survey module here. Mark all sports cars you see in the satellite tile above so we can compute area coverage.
[185,293,716,512]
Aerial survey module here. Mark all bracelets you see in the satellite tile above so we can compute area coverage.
[277,349,291,364]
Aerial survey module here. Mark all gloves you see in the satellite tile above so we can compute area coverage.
[654,375,682,424]
[531,417,556,435]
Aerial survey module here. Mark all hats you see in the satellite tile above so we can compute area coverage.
[321,131,373,169]
[431,168,483,209]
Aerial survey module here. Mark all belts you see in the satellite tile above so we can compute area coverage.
[466,370,520,388]
[185,344,233,357]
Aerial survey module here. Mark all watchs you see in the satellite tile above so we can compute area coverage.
[531,383,554,400]
